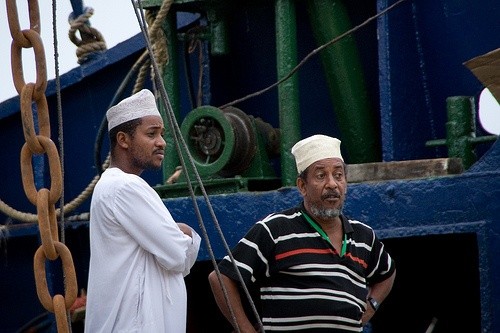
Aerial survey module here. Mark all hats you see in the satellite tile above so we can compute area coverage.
[106,89,160,132]
[291,134,344,177]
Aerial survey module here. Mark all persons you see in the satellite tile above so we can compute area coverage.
[208,134,397,333]
[84,90,201,333]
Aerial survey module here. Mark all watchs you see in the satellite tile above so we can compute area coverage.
[366,296,380,311]
